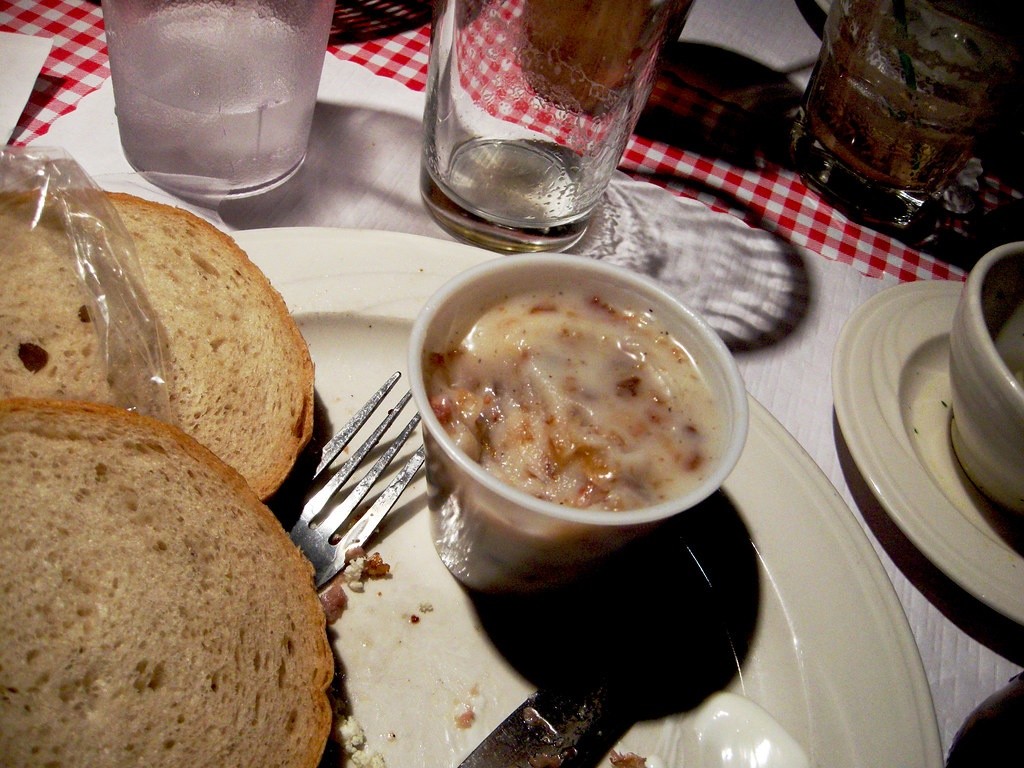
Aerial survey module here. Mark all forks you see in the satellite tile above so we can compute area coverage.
[290,372,426,591]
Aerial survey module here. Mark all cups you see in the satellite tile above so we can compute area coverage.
[950,241,1023,509]
[797,0,1023,232]
[407,252,747,596]
[645,41,799,159]
[101,0,336,202]
[419,1,694,253]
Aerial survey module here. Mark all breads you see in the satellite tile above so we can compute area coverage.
[0,188,313,504]
[0,397,334,768]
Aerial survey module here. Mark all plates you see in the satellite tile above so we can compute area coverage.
[832,281,1023,624]
[222,227,945,768]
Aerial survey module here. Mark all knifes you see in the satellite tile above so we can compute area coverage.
[460,689,636,768]
